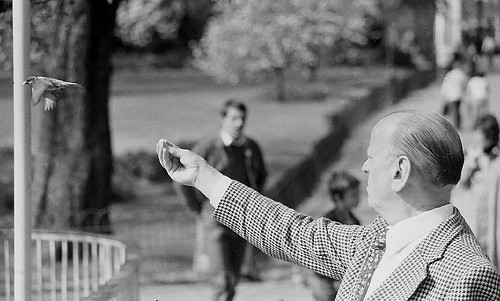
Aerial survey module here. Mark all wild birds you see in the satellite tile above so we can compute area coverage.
[23,75,84,111]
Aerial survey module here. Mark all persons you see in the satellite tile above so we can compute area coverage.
[439,13,500,268]
[174,98,268,301]
[157,109,500,301]
[310,168,364,301]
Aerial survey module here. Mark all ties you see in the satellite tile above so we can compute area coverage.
[349,228,387,301]
